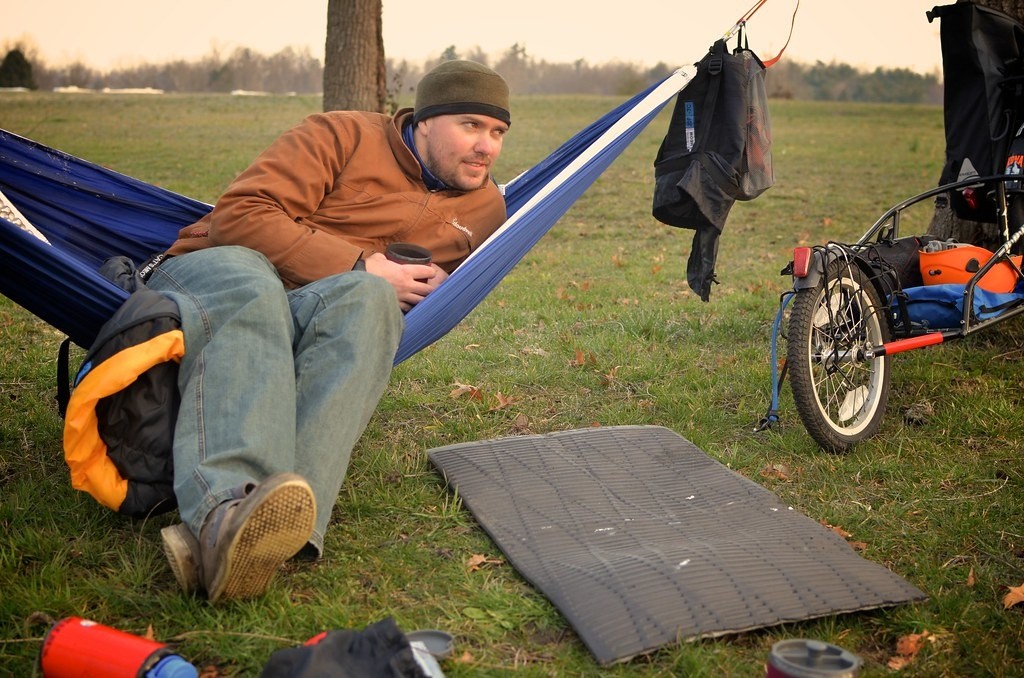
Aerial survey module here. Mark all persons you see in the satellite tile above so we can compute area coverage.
[133,61,515,606]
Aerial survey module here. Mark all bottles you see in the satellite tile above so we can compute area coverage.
[42,616,196,678]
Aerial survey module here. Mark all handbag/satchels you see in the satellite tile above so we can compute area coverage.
[731,21,774,201]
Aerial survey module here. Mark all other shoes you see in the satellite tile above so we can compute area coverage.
[161,522,209,601]
[199,471,318,607]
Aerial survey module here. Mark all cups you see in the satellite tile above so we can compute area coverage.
[384,243,432,284]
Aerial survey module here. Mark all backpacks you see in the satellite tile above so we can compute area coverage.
[654,25,748,303]
[57,289,185,517]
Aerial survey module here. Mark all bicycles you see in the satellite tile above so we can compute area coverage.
[787,154,1024,454]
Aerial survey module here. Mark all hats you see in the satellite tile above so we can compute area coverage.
[413,60,511,129]
